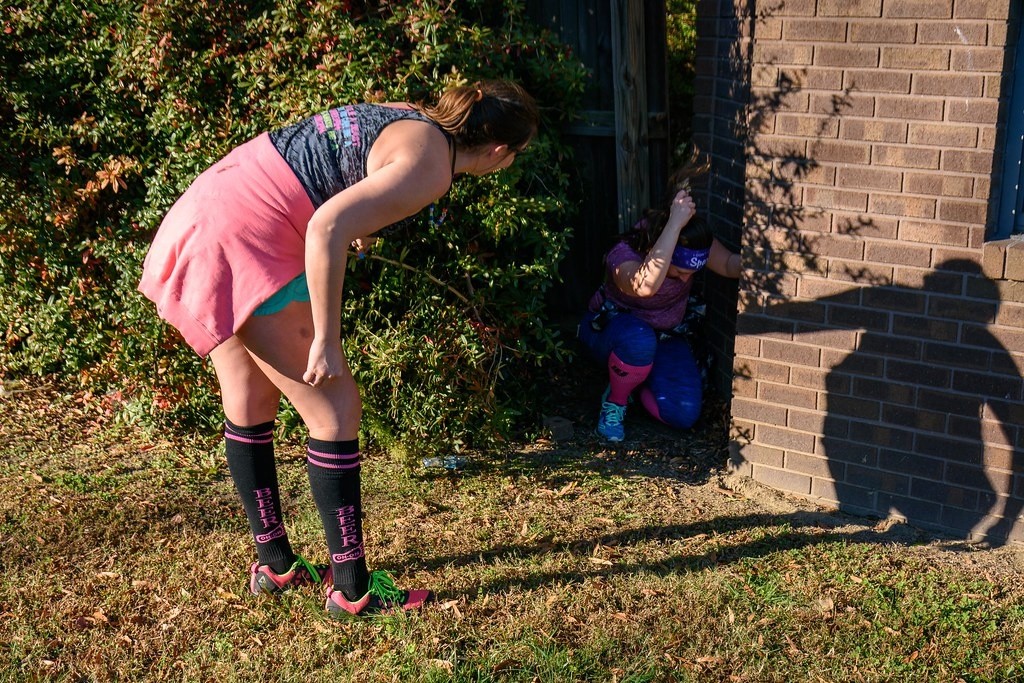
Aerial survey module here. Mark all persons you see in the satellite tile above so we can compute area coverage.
[576,187,742,442]
[134,77,541,616]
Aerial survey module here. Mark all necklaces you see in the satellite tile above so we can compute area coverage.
[429,133,456,235]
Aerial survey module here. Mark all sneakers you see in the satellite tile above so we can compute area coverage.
[324,587,436,624]
[597,385,632,442]
[251,555,334,598]
